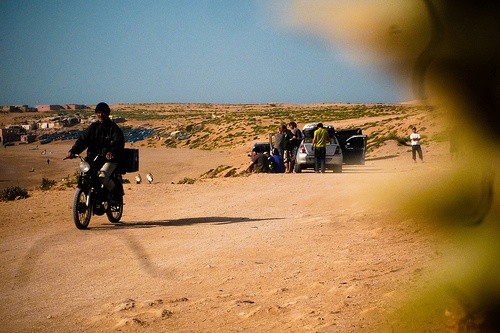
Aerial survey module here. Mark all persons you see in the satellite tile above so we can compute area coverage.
[410,127,425,163]
[447,125,463,160]
[47,159,50,164]
[66,102,125,206]
[245,122,332,173]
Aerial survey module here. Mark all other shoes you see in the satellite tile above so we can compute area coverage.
[315,171,318,173]
[321,171,325,173]
[110,184,120,200]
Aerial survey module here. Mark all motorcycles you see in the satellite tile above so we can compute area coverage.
[63,153,126,229]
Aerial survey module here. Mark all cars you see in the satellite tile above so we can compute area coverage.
[247,141,271,159]
[268,122,368,173]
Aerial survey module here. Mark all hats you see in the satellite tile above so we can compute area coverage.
[95,102,110,112]
[272,148,278,154]
